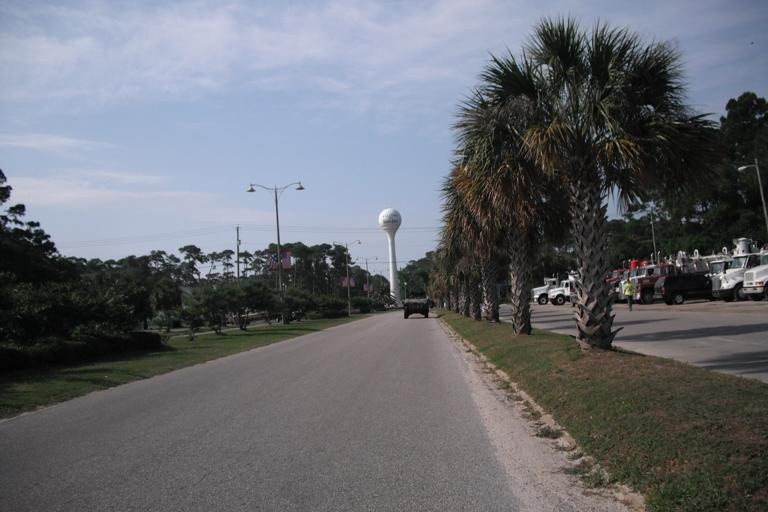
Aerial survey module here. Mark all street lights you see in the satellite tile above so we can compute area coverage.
[637,205,657,261]
[736,158,768,231]
[246,179,307,323]
[355,256,379,297]
[332,240,363,316]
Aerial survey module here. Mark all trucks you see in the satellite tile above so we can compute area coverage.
[743,252,768,301]
[530,237,761,307]
[403,298,430,318]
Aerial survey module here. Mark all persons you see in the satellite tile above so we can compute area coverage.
[623,278,634,311]
